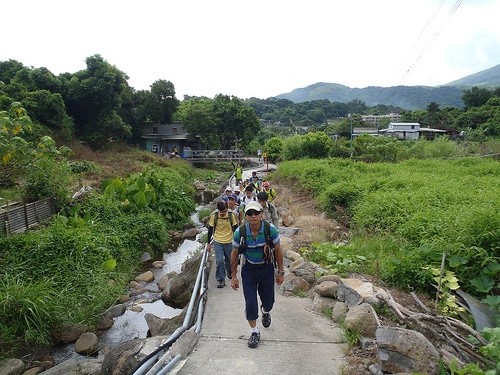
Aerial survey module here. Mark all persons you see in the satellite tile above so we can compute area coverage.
[257,148,262,161]
[174,146,178,154]
[231,201,284,348]
[229,160,243,186]
[207,202,238,288]
[223,172,276,265]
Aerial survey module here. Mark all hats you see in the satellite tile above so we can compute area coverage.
[264,182,269,186]
[234,186,240,192]
[229,193,237,200]
[244,201,263,212]
[225,187,233,191]
[239,180,242,184]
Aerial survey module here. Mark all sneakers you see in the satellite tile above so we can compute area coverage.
[217,280,225,288]
[227,271,232,279]
[261,305,271,328]
[248,332,260,348]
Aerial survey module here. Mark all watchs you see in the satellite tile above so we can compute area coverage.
[278,271,284,275]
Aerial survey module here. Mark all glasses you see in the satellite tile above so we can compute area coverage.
[246,211,263,216]
